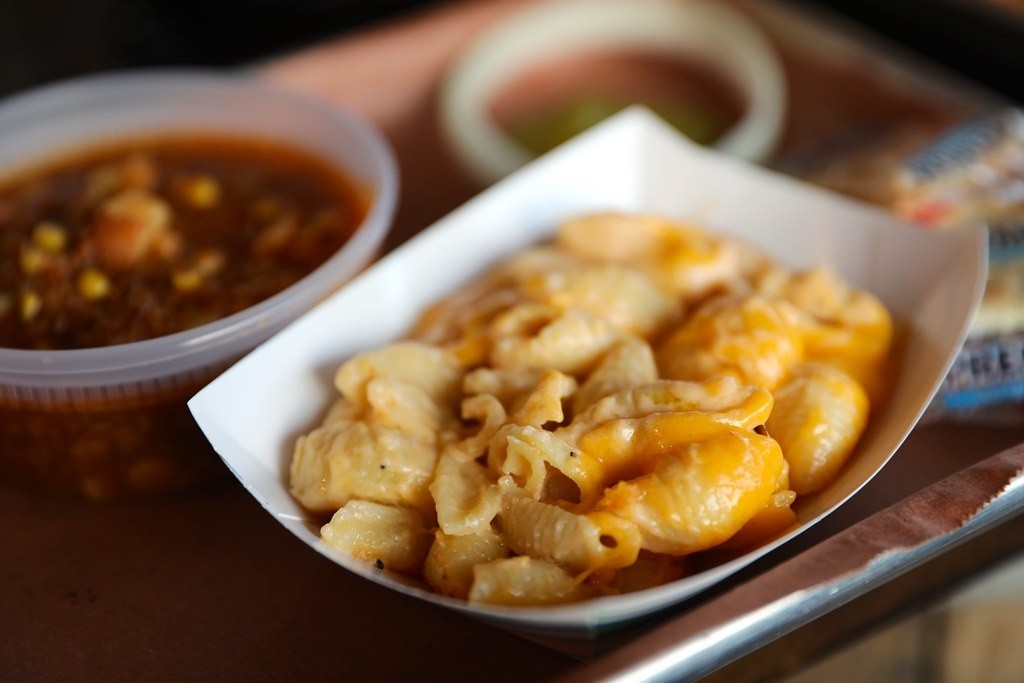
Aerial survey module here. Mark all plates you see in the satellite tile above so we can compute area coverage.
[186,100,990,626]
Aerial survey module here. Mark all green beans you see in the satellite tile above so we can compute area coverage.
[21,163,219,313]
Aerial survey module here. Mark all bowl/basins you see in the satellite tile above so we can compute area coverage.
[0,69,402,510]
[437,1,792,211]
